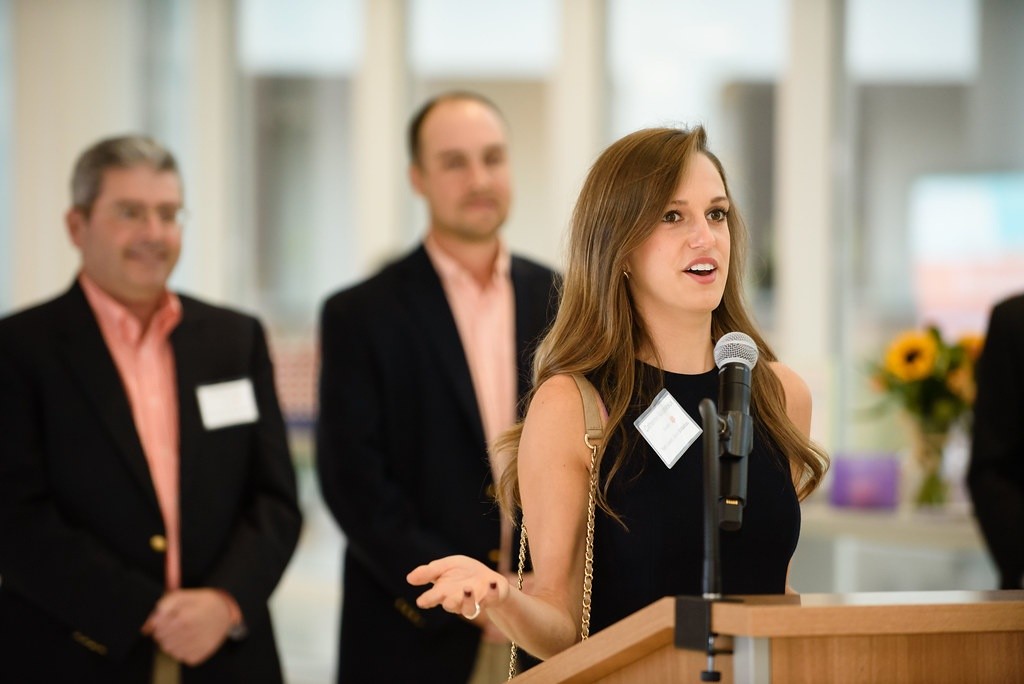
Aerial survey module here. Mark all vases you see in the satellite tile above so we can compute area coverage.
[908,426,954,512]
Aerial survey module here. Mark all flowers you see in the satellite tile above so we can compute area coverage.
[852,317,993,514]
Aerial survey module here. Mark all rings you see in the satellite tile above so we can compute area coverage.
[463,604,480,620]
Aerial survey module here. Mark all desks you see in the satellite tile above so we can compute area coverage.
[800,508,982,591]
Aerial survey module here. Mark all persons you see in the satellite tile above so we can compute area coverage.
[406,124,829,673]
[317,92,566,684]
[0,133,305,684]
[967,293,1024,589]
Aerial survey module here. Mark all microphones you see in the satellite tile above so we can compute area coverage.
[713,332,760,531]
